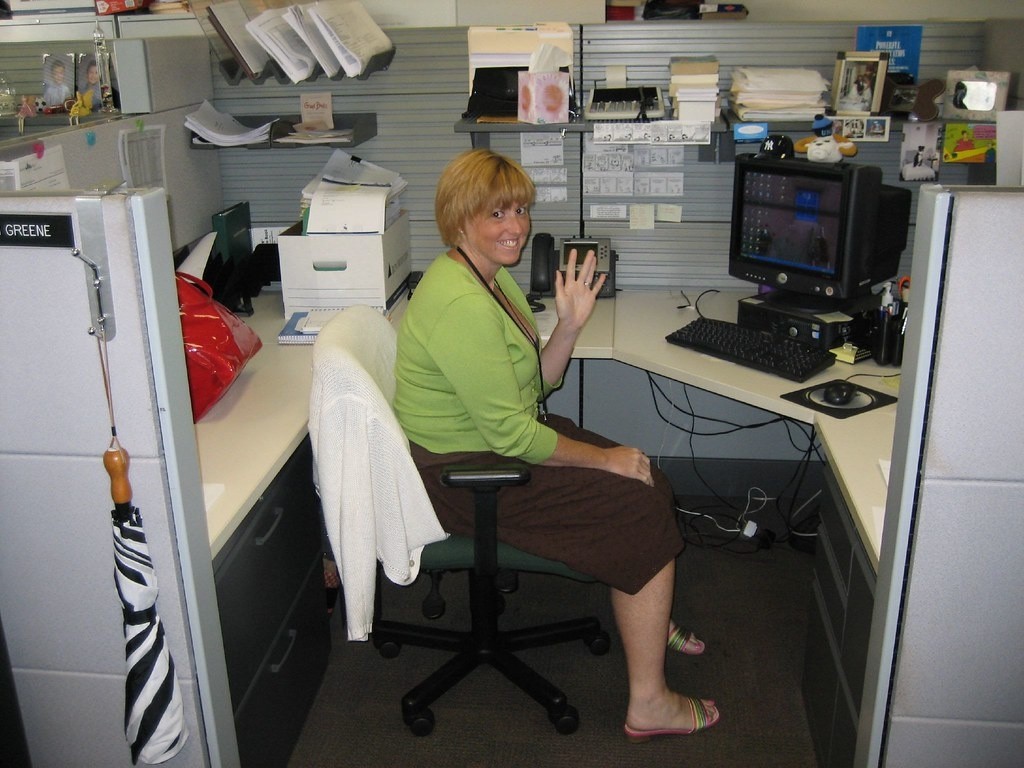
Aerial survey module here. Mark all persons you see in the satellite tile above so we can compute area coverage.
[848,74,871,102]
[68,91,82,127]
[913,145,926,168]
[44,61,72,106]
[394,150,722,743]
[15,97,35,136]
[79,60,102,106]
[870,121,884,135]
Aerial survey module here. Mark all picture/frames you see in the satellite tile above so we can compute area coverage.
[829,51,890,116]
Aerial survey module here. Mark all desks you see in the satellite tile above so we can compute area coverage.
[191,281,904,768]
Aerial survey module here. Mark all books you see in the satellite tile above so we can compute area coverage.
[668,55,722,118]
[728,67,829,122]
[277,305,392,346]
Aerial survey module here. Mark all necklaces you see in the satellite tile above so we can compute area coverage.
[457,247,548,421]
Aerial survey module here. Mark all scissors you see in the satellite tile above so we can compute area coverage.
[899,276,911,304]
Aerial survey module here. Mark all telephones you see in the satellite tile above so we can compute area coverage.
[532,232,617,300]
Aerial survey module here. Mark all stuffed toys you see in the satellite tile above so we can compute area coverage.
[793,114,858,163]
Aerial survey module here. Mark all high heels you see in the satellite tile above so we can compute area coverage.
[624,696,720,744]
[667,622,705,655]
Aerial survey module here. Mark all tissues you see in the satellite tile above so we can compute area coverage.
[517,44,574,125]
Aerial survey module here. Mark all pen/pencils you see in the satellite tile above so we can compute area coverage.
[879,300,908,319]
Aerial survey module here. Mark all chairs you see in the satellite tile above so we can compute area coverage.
[307,306,612,736]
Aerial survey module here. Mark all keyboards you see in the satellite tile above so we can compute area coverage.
[665,317,837,384]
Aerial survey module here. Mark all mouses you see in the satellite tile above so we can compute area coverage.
[824,380,858,405]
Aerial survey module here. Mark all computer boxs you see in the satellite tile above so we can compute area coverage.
[737,292,885,357]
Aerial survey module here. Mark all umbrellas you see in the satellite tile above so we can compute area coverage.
[102,448,190,765]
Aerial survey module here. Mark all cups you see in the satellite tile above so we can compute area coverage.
[868,315,904,367]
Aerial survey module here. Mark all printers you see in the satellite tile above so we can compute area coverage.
[461,21,573,121]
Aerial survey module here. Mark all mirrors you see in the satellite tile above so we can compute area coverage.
[943,69,1015,122]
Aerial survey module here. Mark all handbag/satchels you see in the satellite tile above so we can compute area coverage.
[175,271,262,424]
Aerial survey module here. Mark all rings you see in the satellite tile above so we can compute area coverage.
[585,282,591,286]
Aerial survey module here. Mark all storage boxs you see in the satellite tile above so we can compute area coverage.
[277,210,410,320]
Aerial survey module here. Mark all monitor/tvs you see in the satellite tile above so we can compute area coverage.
[729,153,912,315]
